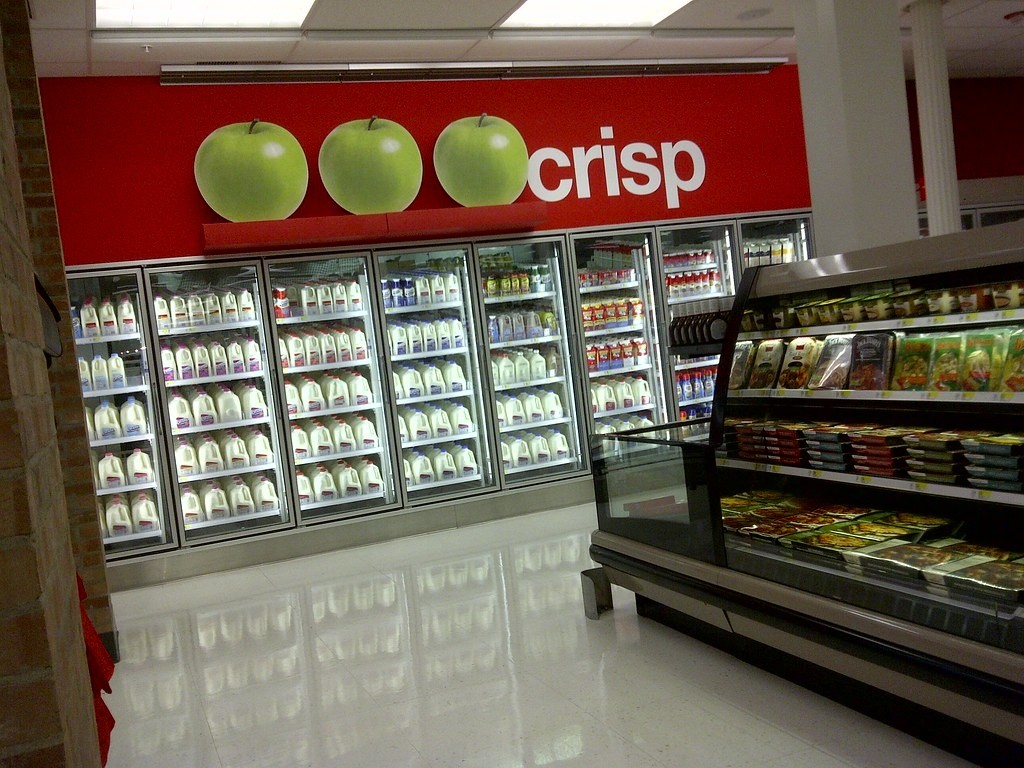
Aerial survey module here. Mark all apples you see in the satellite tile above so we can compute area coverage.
[191,123,308,222]
[433,116,531,208]
[318,118,422,214]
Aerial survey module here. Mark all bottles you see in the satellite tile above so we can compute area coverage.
[152,288,282,526]
[664,248,734,317]
[672,357,718,433]
[479,260,574,472]
[269,276,385,506]
[738,233,798,270]
[375,265,481,489]
[65,291,160,538]
[573,249,655,455]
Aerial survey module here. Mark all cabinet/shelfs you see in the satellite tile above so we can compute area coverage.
[712,219,1024,768]
